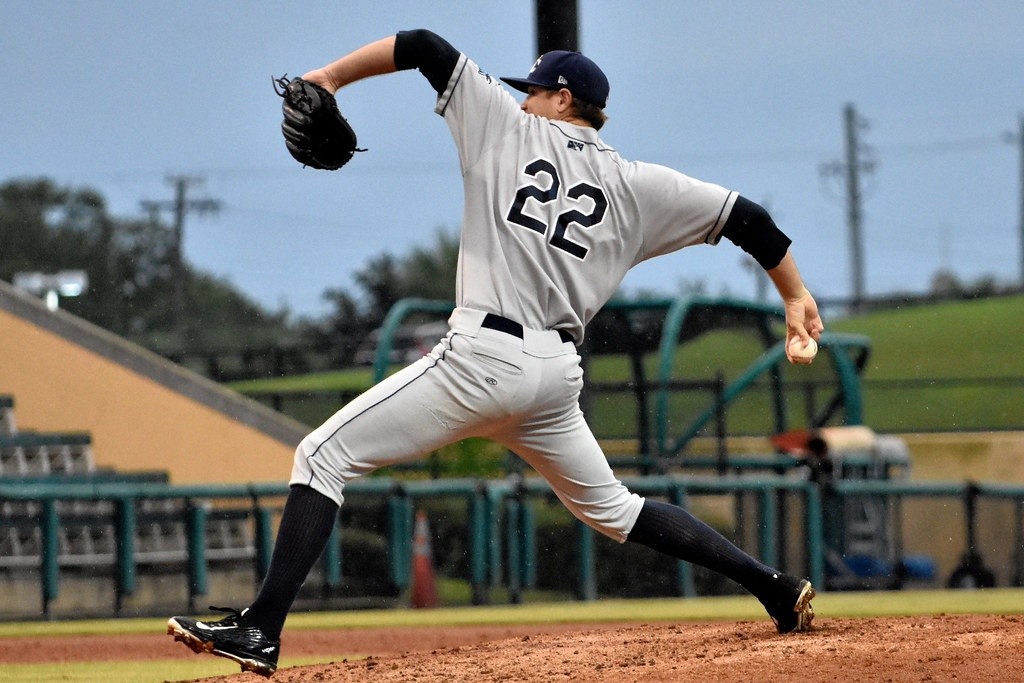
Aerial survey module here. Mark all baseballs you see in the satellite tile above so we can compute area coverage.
[788,334,818,363]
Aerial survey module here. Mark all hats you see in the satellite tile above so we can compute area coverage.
[499,51,609,107]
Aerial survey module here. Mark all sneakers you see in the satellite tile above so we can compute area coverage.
[166,606,280,678]
[768,569,815,634]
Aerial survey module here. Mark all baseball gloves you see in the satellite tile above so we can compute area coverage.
[280,75,358,172]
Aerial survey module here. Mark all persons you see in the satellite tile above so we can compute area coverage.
[166,29,825,677]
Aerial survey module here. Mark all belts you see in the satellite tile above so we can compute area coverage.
[482,312,575,343]
